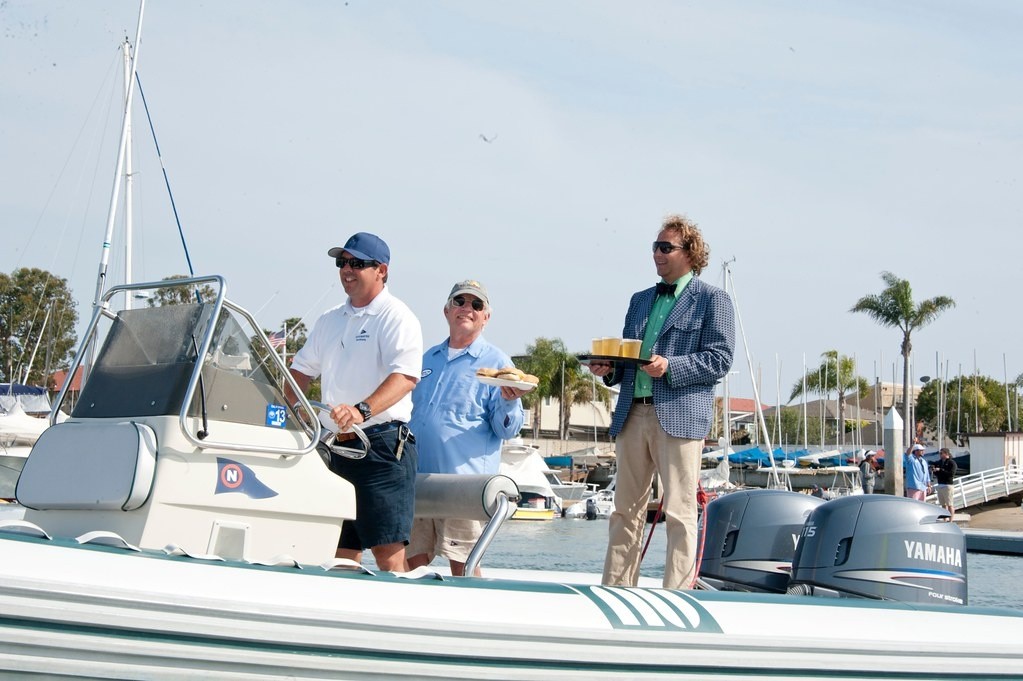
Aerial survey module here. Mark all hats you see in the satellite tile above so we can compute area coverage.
[327,232,389,266]
[447,280,488,303]
[865,450,877,457]
[913,444,925,451]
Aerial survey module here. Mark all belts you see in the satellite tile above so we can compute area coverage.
[335,421,400,442]
[632,396,654,405]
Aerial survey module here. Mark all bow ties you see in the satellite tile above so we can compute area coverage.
[656,282,677,294]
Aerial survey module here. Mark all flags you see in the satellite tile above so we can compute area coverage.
[269,331,286,346]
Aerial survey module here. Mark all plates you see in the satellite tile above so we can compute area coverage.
[477,376,538,393]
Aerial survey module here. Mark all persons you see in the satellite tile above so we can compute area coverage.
[581,217,735,589]
[932,448,957,523]
[285,232,423,573]
[406,280,534,576]
[904,437,931,502]
[858,450,880,494]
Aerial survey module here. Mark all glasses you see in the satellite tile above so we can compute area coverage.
[652,241,689,254]
[448,296,487,311]
[336,257,379,268]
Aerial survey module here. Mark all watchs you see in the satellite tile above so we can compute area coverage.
[355,402,371,422]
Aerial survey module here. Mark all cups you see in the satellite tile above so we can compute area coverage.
[592,338,643,358]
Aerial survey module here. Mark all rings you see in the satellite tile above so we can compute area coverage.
[510,394,514,398]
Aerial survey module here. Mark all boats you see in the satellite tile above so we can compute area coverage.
[717,448,765,467]
[818,450,862,467]
[496,437,563,519]
[0,383,52,418]
[565,472,617,520]
[928,451,970,474]
[845,449,885,470]
[875,451,943,468]
[544,446,614,466]
[0,274,1023,681]
[572,452,616,466]
[762,449,811,466]
[702,447,735,463]
[744,448,787,466]
[798,449,840,467]
[541,468,588,510]
[728,462,860,487]
[0,402,70,498]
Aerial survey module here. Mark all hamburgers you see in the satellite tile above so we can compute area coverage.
[477,367,539,384]
